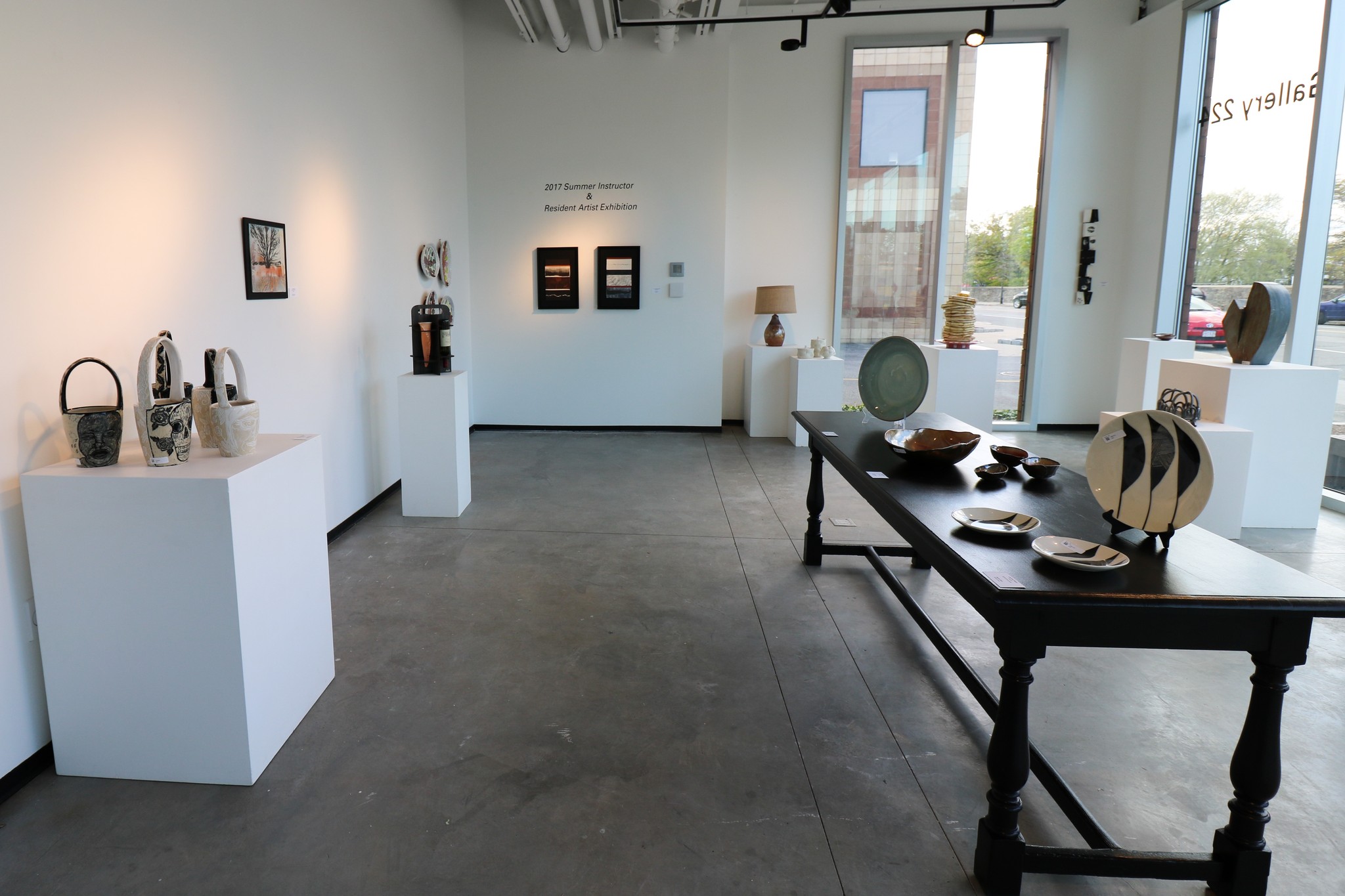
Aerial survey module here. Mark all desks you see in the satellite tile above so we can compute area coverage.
[745,344,802,438]
[1156,359,1339,528]
[1116,337,1196,411]
[788,356,844,447]
[790,410,1345,896]
[396,369,471,517]
[920,344,998,434]
[21,432,335,786]
[1100,411,1254,540]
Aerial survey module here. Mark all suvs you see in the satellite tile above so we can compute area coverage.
[1192,286,1207,299]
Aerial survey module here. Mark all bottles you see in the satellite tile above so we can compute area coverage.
[438,319,451,368]
[797,336,826,359]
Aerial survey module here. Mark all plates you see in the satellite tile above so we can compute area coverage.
[858,336,929,422]
[951,507,1041,536]
[420,240,454,324]
[1152,333,1176,341]
[934,339,984,349]
[1086,409,1215,533]
[1032,535,1130,572]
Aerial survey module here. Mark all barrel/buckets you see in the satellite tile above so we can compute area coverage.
[59,330,260,468]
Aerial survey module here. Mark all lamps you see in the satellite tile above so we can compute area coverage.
[754,285,797,347]
[780,19,807,52]
[965,9,994,47]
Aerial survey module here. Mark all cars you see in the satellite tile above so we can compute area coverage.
[1012,288,1029,308]
[1188,295,1229,349]
[1317,294,1345,324]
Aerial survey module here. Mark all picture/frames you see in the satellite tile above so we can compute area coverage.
[242,217,288,300]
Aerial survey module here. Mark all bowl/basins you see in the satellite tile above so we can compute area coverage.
[990,444,1029,466]
[884,428,982,466]
[1020,457,1062,480]
[974,463,1008,481]
